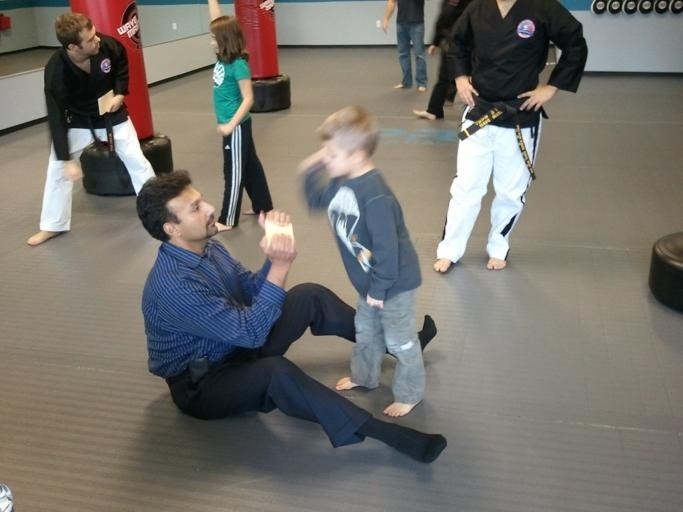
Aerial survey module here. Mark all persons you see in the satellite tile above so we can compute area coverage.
[25,13,158,248]
[432,1,588,275]
[292,104,427,419]
[134,168,447,465]
[381,0,431,94]
[208,0,274,233]
[412,0,471,123]
